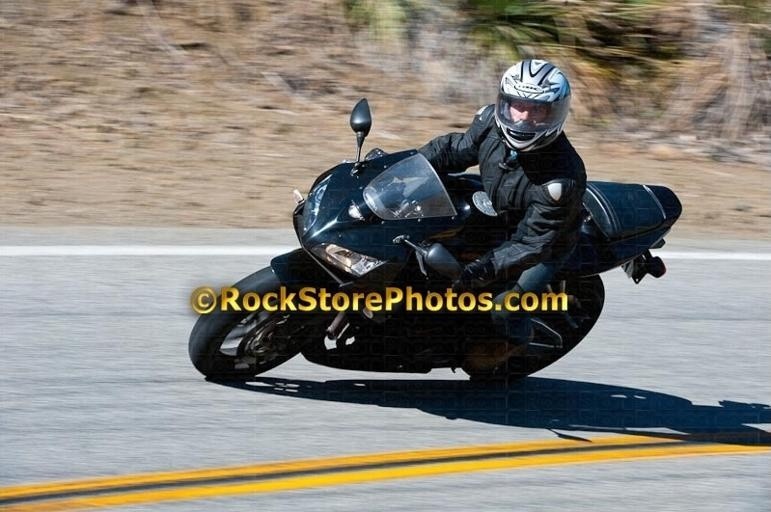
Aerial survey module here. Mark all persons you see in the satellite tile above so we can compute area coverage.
[364,57,588,371]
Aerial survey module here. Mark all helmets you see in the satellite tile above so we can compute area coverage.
[493,58,572,154]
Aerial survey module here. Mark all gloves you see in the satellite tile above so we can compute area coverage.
[452,260,495,292]
[364,147,386,161]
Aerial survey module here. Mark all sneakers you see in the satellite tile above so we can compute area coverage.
[468,328,534,368]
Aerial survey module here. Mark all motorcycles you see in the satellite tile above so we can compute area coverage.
[188,98,682,389]
